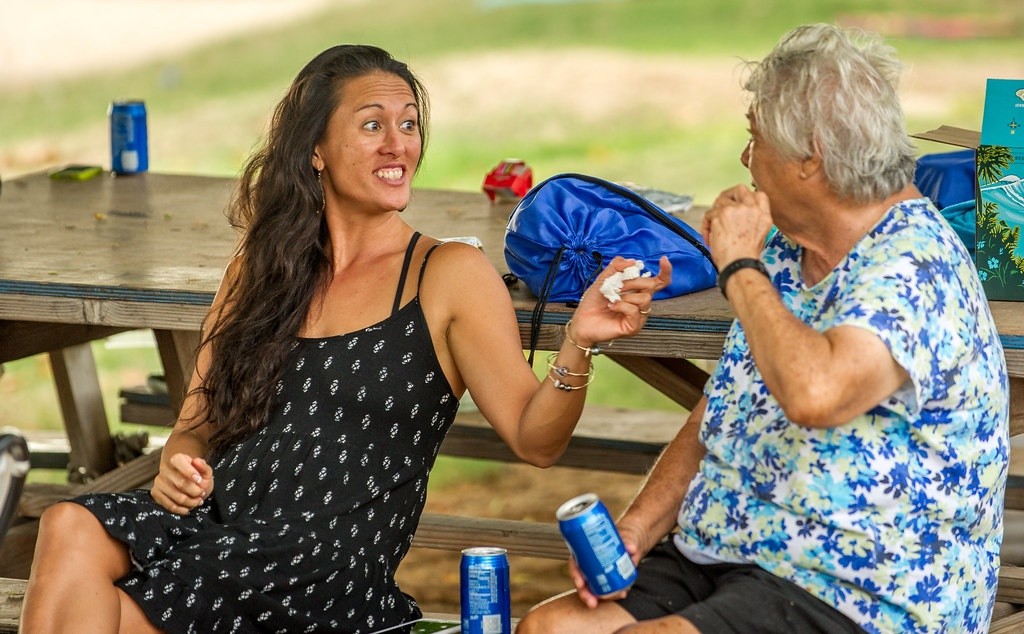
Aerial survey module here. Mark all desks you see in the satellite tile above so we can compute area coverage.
[1,167,1023,481]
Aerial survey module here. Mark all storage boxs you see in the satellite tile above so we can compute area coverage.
[908,78,1023,303]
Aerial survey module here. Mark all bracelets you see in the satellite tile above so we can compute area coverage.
[547,320,614,391]
[719,257,771,298]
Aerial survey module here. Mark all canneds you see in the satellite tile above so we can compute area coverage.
[108,100,149,174]
[554,492,639,597]
[458,547,512,634]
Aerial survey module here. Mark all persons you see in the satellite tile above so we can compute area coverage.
[514,24,1010,634]
[19,45,672,634]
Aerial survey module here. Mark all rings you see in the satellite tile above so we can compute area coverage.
[641,308,652,314]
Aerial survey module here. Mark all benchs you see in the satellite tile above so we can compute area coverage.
[1,373,1024,634]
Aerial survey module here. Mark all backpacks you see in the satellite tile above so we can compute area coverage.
[503,173,718,368]
